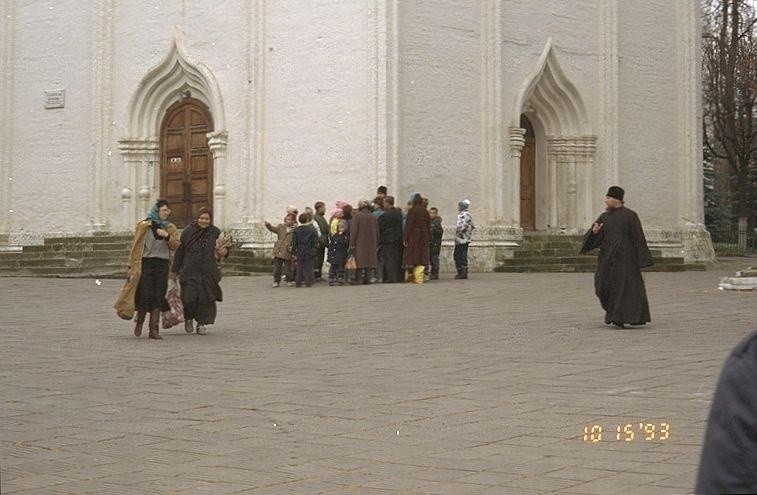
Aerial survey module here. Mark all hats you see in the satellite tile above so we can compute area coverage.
[606,186,624,200]
[459,199,470,210]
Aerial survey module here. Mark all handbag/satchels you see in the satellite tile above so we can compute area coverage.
[344,255,356,271]
[403,265,425,284]
[163,286,186,328]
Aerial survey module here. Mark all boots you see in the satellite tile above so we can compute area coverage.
[455,265,467,279]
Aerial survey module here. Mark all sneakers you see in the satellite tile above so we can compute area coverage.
[273,275,321,287]
[184,319,193,332]
[196,325,207,335]
[328,271,391,286]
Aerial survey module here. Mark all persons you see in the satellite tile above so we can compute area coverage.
[578,187,653,329]
[265,186,443,288]
[453,199,473,279]
[169,207,230,335]
[127,199,180,340]
[692,329,757,494]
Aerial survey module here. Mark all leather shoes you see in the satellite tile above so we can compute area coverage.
[134,320,141,336]
[149,330,162,339]
